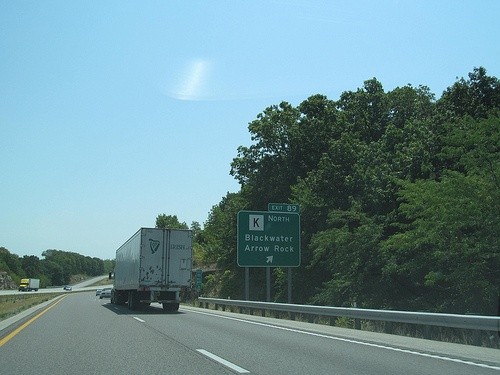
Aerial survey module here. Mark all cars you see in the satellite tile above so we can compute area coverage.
[100,288,112,298]
[64,286,68,290]
[66,287,72,290]
[96,289,102,296]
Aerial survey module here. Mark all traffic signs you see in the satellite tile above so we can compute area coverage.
[237,204,300,266]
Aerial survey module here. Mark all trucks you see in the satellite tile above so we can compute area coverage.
[109,230,191,312]
[18,278,39,292]
